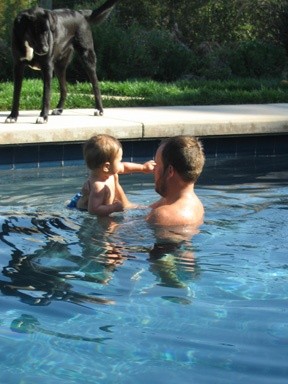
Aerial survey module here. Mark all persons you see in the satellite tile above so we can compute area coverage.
[64,136,206,245]
[64,217,202,305]
[76,133,157,216]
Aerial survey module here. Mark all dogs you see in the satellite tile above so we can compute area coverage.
[2,0,116,124]
